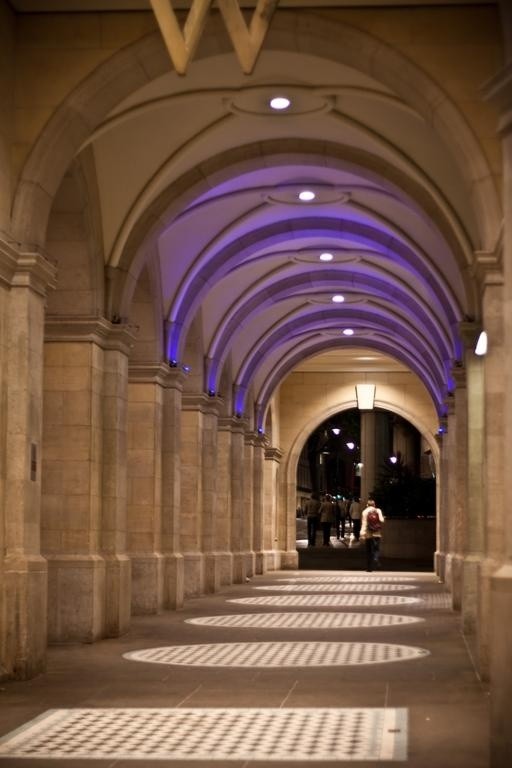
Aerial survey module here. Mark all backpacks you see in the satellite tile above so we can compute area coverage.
[367,510,382,531]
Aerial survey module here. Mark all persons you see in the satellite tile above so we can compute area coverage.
[305,492,360,551]
[358,499,387,572]
